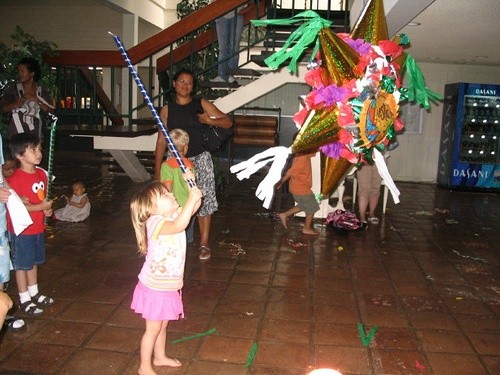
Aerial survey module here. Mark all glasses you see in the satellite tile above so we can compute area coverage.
[176,79,193,88]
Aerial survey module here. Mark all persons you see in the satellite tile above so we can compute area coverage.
[209,0,248,82]
[160,128,196,244]
[276,130,320,235]
[0,57,53,239]
[51,181,91,222]
[0,290,14,331]
[153,71,233,260]
[132,168,203,375]
[356,150,388,227]
[0,134,26,331]
[5,133,54,317]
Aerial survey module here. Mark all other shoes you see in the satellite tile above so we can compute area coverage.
[198,246,210,259]
[369,215,380,224]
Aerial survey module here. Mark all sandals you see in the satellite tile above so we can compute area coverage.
[30,294,55,305]
[18,300,44,316]
[3,316,26,331]
